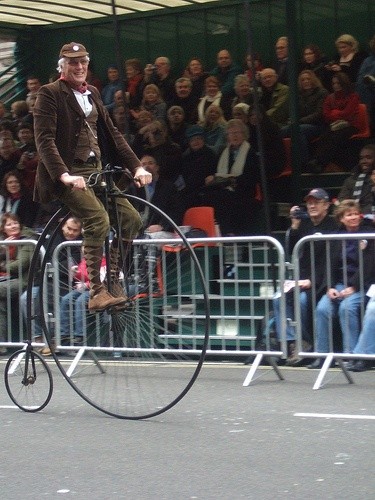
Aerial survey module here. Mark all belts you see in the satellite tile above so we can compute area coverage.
[73,155,97,164]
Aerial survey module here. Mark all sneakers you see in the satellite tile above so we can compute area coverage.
[107,283,134,311]
[88,287,125,314]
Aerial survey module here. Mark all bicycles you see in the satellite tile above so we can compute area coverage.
[4,166,211,419]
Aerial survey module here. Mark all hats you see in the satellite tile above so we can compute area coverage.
[305,187,329,201]
[59,42,89,58]
[186,125,205,138]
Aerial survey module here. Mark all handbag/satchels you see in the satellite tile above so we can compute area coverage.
[330,119,349,130]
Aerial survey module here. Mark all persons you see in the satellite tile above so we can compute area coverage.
[243,52,265,82]
[274,36,289,84]
[232,102,251,124]
[296,70,329,167]
[275,143,375,371]
[197,76,232,120]
[175,126,218,186]
[140,155,177,231]
[197,103,226,156]
[230,74,260,109]
[303,44,333,91]
[198,119,260,237]
[247,104,287,179]
[355,37,375,111]
[209,48,242,96]
[302,72,360,174]
[0,56,206,358]
[322,34,369,91]
[256,68,290,128]
[33,42,153,313]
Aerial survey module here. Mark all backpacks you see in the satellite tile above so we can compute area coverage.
[247,310,285,366]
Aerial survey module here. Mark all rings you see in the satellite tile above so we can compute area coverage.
[74,183,77,185]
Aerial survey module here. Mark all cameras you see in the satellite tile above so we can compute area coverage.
[294,210,309,220]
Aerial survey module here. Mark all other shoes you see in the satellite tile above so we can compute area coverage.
[307,357,335,369]
[32,337,42,353]
[349,359,372,372]
[67,342,80,356]
[41,348,52,356]
[0,346,7,354]
[342,359,356,370]
[286,348,306,365]
[224,264,234,279]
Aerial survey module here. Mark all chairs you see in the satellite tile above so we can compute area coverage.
[352,103,370,138]
[133,206,216,300]
[274,138,292,179]
